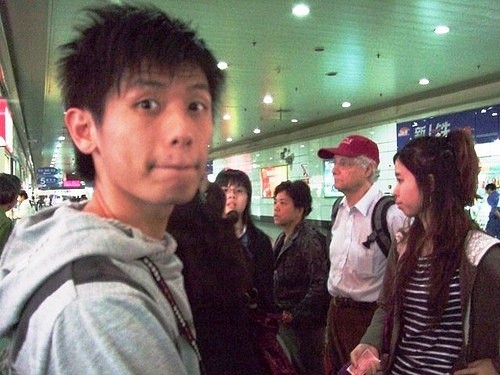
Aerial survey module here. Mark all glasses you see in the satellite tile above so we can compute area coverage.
[221,186,247,196]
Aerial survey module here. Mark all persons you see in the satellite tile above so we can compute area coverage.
[484,184,500,239]
[166,168,271,375]
[10,189,31,220]
[271,180,332,375]
[318,135,418,375]
[349,126,500,375]
[214,169,294,329]
[0,0,226,375]
[0,172,22,255]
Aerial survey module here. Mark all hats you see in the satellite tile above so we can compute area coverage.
[317,134,380,168]
